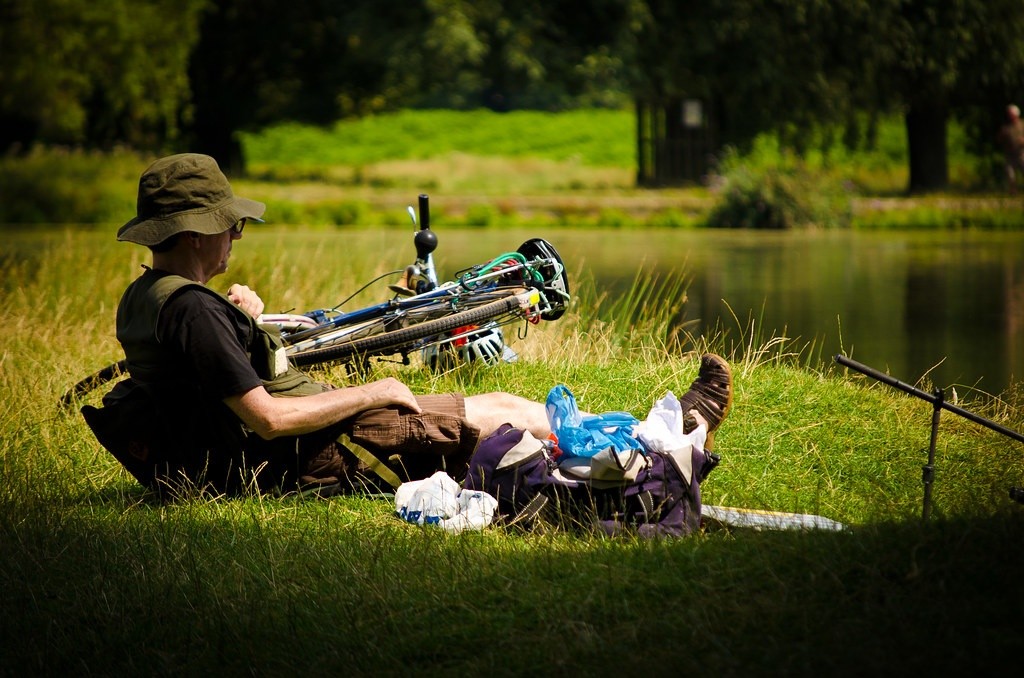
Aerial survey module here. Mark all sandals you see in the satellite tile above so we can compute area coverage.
[680,353,732,451]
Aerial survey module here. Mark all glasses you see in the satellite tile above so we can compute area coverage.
[232,218,247,233]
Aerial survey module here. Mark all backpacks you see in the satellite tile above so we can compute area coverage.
[78,272,288,501]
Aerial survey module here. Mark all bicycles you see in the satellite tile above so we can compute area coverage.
[252,193,573,374]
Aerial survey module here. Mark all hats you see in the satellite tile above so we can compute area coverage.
[116,154,266,246]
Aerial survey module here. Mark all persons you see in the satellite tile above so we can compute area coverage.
[116,153,733,498]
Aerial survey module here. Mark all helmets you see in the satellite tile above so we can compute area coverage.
[425,321,504,375]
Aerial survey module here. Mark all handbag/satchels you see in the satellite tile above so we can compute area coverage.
[461,422,706,541]
[394,471,499,533]
[546,385,645,458]
